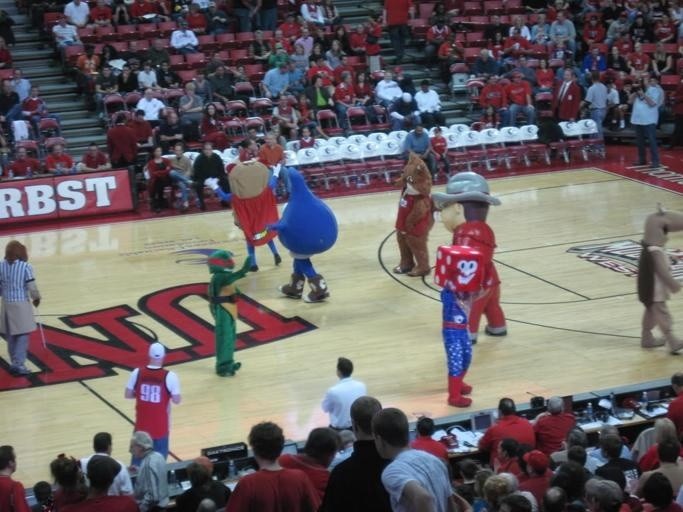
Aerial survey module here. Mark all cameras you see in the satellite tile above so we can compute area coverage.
[630,85,640,94]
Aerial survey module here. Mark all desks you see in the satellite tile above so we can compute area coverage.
[447,397,673,464]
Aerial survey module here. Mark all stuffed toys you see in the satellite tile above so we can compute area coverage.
[432,173,510,337]
[391,155,436,276]
[268,169,339,303]
[637,202,683,354]
[208,249,249,376]
[204,154,282,272]
[433,244,493,407]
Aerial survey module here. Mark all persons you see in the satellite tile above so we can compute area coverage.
[124,343,181,461]
[0,241,42,374]
[1,0,682,209]
[0,357,682,512]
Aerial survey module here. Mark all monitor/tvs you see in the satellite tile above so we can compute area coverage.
[279,443,299,457]
[471,411,495,433]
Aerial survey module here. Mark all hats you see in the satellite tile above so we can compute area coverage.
[86,455,122,478]
[149,343,165,358]
[132,432,154,448]
[524,450,549,470]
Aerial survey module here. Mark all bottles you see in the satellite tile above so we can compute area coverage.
[228,460,235,482]
[586,402,593,423]
[608,392,616,414]
[169,469,177,493]
[639,391,648,414]
